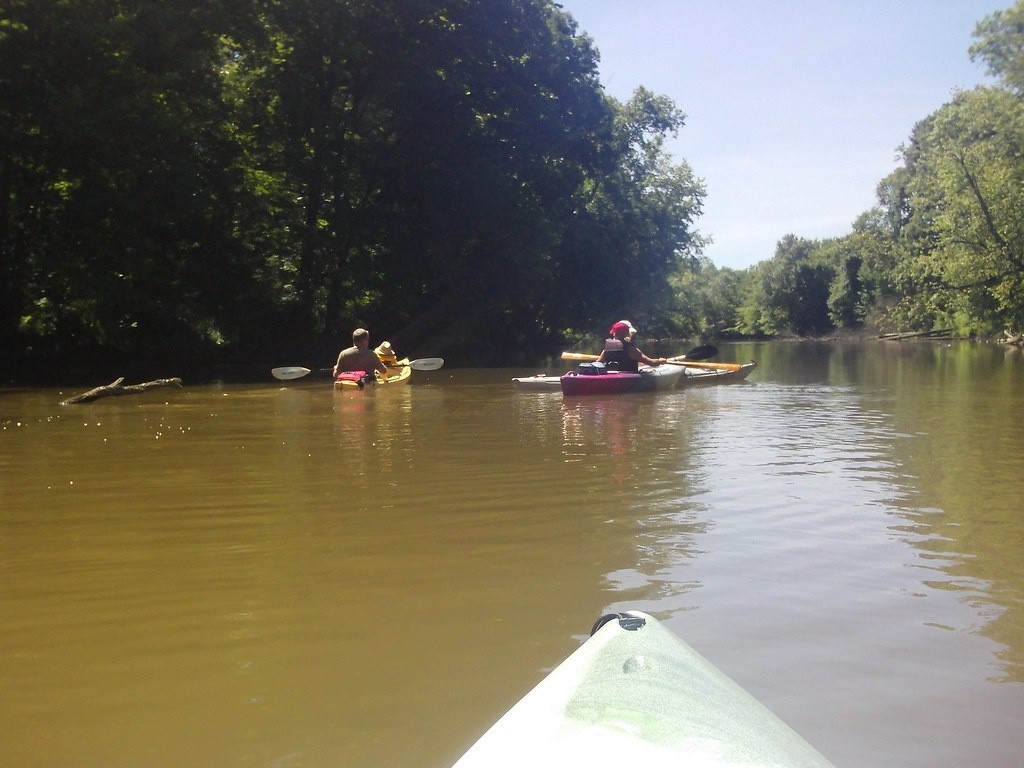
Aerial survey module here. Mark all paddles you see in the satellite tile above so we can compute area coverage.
[271,358,444,381]
[559,351,742,373]
[666,344,720,363]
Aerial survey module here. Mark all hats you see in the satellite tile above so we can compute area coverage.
[610,320,637,338]
[374,341,395,355]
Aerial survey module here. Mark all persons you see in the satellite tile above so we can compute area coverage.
[595,321,667,372]
[332,328,405,380]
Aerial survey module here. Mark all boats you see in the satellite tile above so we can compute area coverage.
[334,356,411,391]
[512,363,756,391]
[558,363,686,396]
